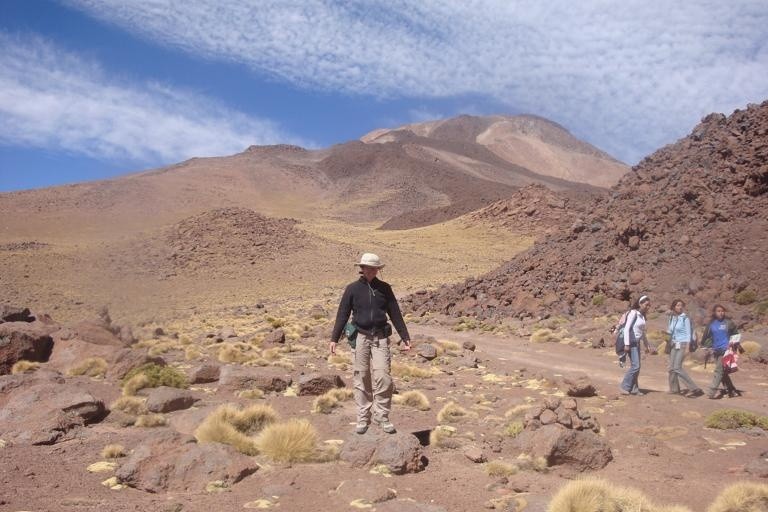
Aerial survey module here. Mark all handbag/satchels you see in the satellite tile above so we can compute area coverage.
[666,339,672,354]
[721,350,737,373]
[616,324,636,352]
[344,323,357,348]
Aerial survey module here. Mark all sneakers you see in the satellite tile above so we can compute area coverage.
[635,390,643,395]
[731,390,738,396]
[356,419,371,433]
[373,417,394,432]
[685,388,701,397]
[619,385,629,395]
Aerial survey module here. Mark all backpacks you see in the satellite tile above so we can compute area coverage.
[683,317,698,352]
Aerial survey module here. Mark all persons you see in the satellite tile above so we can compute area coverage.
[701,304,744,399]
[661,300,702,397]
[619,295,651,396]
[330,253,413,434]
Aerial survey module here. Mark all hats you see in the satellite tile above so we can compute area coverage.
[354,253,385,270]
